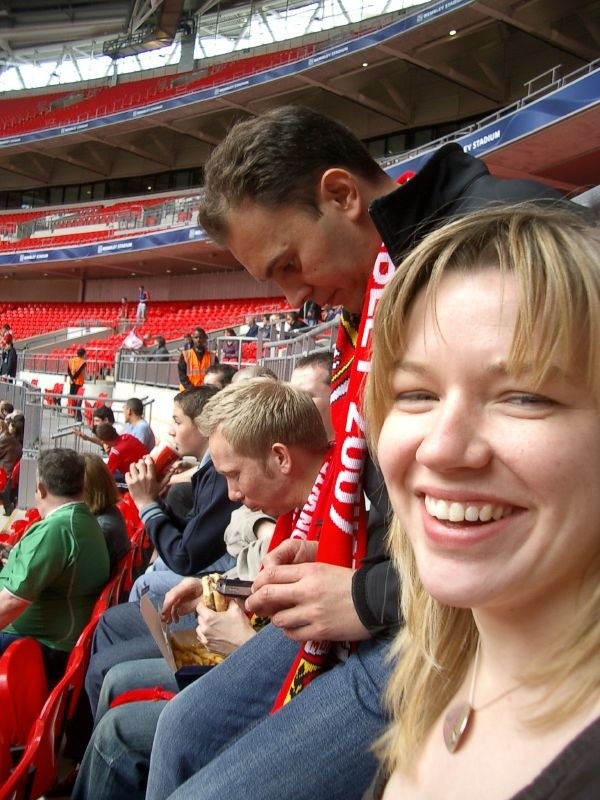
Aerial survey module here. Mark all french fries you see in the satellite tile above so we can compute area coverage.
[171,637,227,669]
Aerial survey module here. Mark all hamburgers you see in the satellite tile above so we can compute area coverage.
[201,573,230,612]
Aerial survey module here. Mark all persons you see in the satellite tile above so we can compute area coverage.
[147,100,584,800]
[0,340,18,384]
[123,397,155,455]
[0,323,14,362]
[164,362,235,518]
[96,423,149,475]
[81,453,132,583]
[136,285,150,323]
[122,382,226,599]
[0,447,112,689]
[71,352,333,717]
[357,195,599,800]
[177,328,221,394]
[67,348,87,422]
[145,378,332,799]
[150,299,346,361]
[72,406,124,457]
[117,296,128,332]
[84,366,278,718]
[0,400,25,484]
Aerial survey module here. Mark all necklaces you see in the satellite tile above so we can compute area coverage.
[442,629,523,753]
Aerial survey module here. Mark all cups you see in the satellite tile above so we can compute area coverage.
[148,439,183,483]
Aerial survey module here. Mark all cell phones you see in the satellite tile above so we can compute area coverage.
[215,579,253,598]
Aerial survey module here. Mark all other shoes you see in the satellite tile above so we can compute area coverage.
[44,762,81,800]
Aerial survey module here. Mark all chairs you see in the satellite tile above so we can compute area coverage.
[0,496,152,800]
[0,298,293,378]
